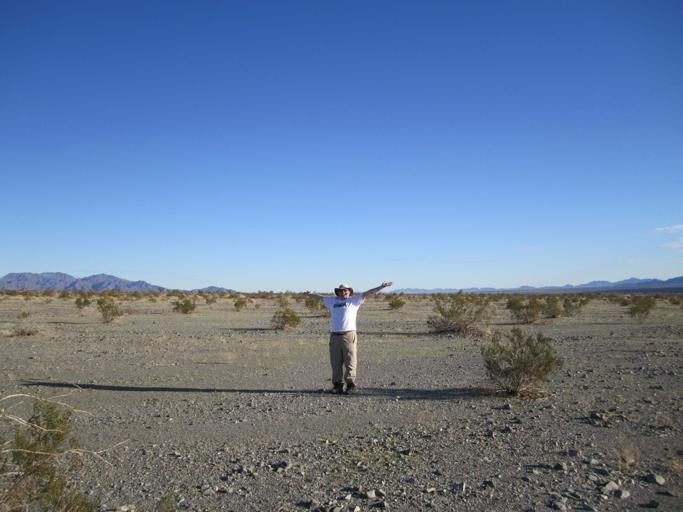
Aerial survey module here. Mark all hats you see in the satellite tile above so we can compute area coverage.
[334,284,352,297]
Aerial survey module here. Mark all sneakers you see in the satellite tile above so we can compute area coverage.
[345,383,353,394]
[331,383,342,393]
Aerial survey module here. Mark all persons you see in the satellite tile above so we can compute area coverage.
[304,281,392,394]
